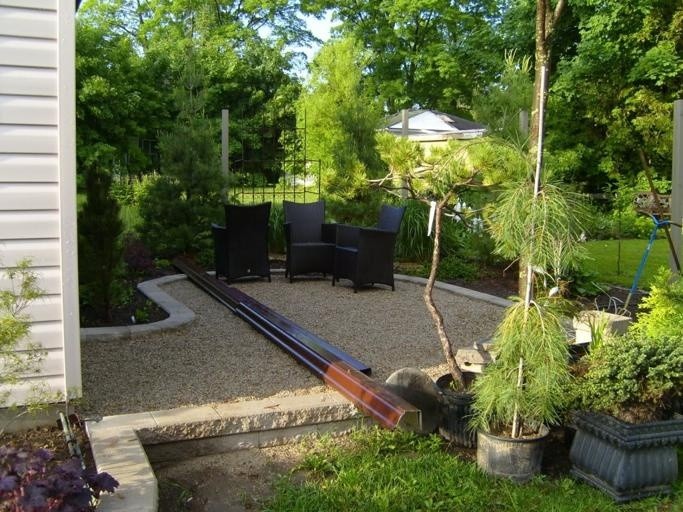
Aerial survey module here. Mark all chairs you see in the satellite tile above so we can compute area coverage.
[211,198,407,294]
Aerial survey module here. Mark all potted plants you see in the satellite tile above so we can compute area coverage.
[328,49,683,505]
